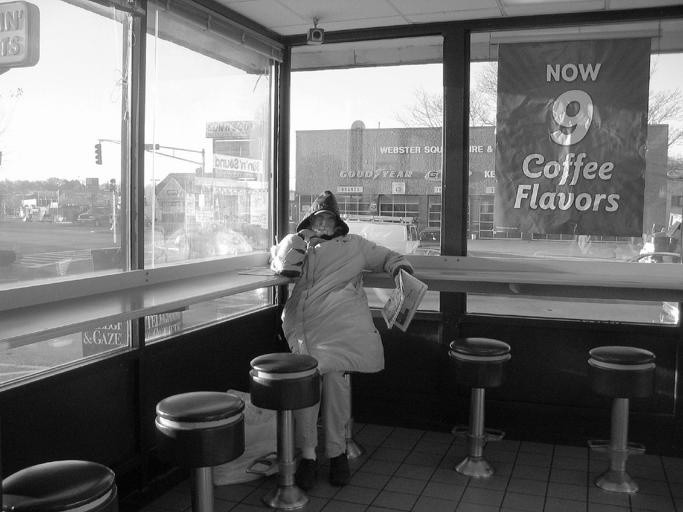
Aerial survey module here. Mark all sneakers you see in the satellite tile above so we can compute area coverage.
[294,458,318,490]
[330,451,351,486]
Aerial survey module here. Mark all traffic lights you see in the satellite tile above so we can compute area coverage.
[94,145,102,164]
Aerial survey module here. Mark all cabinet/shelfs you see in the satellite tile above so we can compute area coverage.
[350,309,447,435]
[142,306,282,499]
[0,348,146,511]
[462,317,683,461]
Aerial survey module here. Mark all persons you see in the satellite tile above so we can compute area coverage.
[270,189,415,489]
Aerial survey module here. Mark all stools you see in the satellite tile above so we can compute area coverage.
[446,336,512,479]
[587,345,660,495]
[248,353,321,509]
[0,459,120,512]
[154,391,245,512]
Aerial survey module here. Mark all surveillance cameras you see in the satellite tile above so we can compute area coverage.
[306,26,325,46]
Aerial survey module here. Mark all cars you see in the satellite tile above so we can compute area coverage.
[154,227,250,262]
[418,227,439,248]
[627,252,682,264]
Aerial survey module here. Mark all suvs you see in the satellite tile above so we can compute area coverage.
[344,216,421,256]
